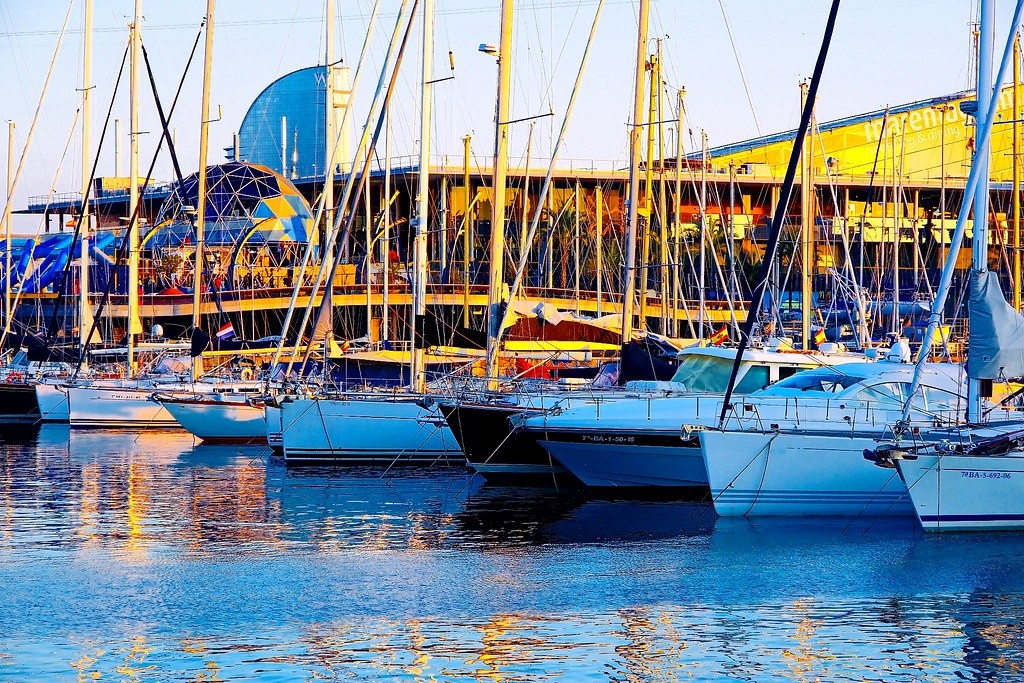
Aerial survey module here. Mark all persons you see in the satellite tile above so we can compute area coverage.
[138,266,314,287]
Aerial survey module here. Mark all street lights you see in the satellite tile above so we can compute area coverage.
[929,105,955,323]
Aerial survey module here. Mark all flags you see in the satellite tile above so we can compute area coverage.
[215,321,235,340]
[709,323,827,346]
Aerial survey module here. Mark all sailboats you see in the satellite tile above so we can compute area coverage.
[0,0,1024,532]
